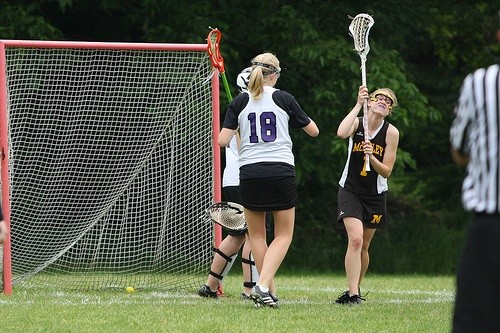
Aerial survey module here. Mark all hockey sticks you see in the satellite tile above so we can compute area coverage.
[204,201,248,230]
[349,12,375,172]
[207,29,234,102]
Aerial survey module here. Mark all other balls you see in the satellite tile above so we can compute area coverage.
[125,286,135,295]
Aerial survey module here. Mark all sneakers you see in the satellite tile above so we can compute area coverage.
[349,296,363,305]
[198,284,222,299]
[239,292,253,300]
[337,292,348,304]
[251,285,277,308]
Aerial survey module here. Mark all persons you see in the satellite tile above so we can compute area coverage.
[199,66,258,300]
[0,202,8,246]
[218,53,319,306]
[337,85,399,302]
[451,27,500,333]
